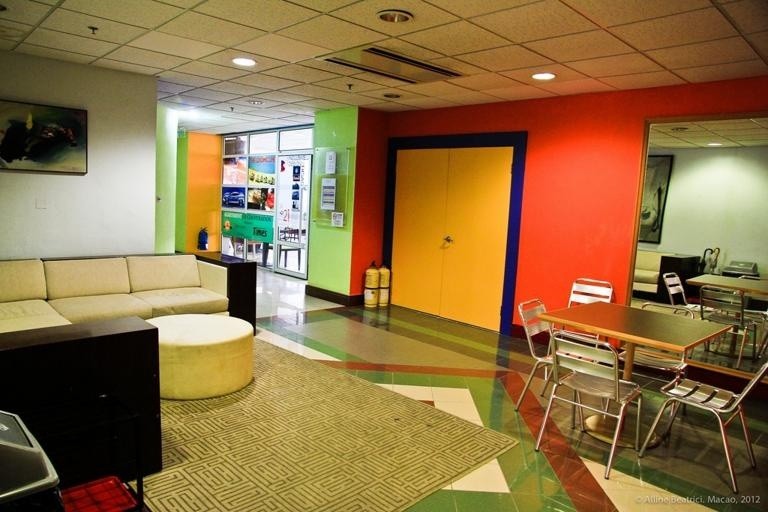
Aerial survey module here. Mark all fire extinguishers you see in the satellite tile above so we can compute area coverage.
[197,226,209,249]
[365,260,391,308]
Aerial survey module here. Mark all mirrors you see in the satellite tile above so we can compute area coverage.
[623,121,768,377]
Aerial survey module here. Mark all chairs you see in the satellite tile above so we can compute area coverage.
[658,269,768,371]
[617,301,695,417]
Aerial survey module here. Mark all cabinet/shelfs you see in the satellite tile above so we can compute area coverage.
[0,315,163,512]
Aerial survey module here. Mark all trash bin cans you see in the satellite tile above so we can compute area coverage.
[0,410,60,512]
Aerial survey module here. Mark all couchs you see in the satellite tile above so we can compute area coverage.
[632,246,703,302]
[1,249,259,340]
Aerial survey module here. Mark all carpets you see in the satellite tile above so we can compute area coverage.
[118,340,520,512]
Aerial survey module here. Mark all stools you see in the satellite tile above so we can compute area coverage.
[142,312,257,402]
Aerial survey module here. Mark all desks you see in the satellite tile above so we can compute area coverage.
[262,240,306,272]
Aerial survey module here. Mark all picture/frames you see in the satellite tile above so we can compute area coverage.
[0,96,90,180]
[637,153,675,244]
